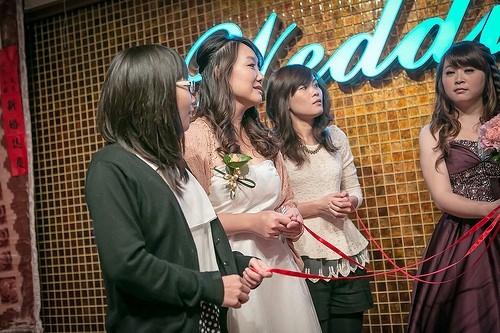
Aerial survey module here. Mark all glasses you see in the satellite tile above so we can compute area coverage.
[176,82,195,94]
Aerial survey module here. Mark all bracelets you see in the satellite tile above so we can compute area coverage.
[294,226,305,239]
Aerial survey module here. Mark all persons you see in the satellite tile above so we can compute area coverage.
[405,40,500,333]
[84,43,273,333]
[265,65,375,333]
[180,34,323,333]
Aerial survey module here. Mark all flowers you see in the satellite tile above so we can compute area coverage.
[477,112,500,161]
[213,153,256,199]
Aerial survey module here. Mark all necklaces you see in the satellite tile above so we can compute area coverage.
[300,143,324,154]
